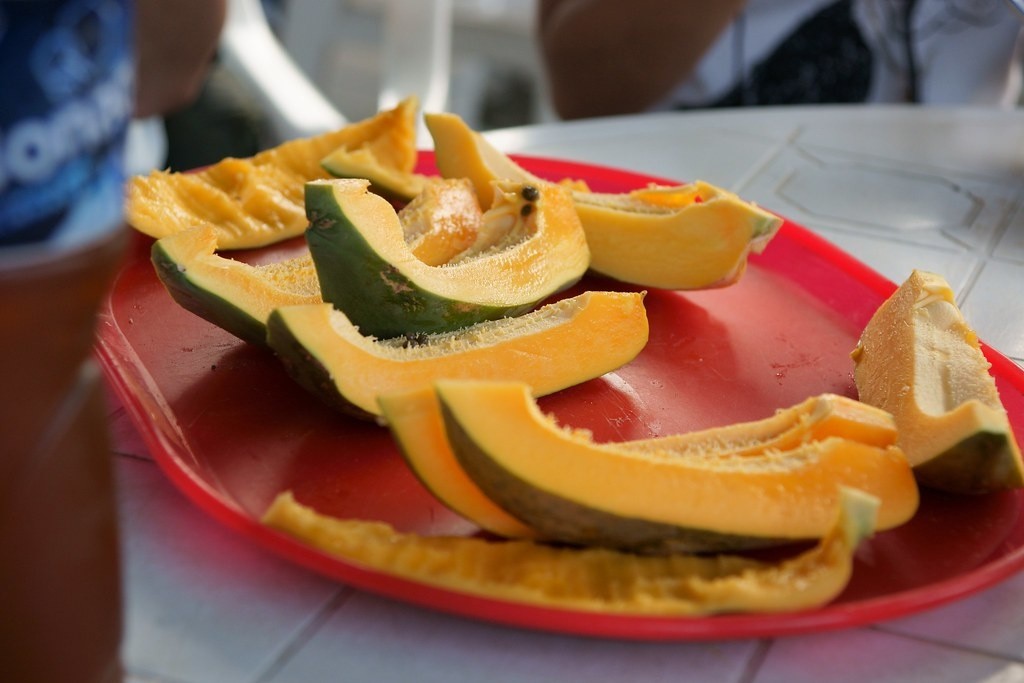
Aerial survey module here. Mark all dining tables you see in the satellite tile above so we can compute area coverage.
[108,104,1024,682]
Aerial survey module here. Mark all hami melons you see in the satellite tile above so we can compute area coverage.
[150,112,1024,555]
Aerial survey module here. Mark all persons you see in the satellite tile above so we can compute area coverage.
[0,0,227,248]
[533,0,1024,123]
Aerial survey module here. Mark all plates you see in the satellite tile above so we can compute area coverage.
[89,151,1024,643]
